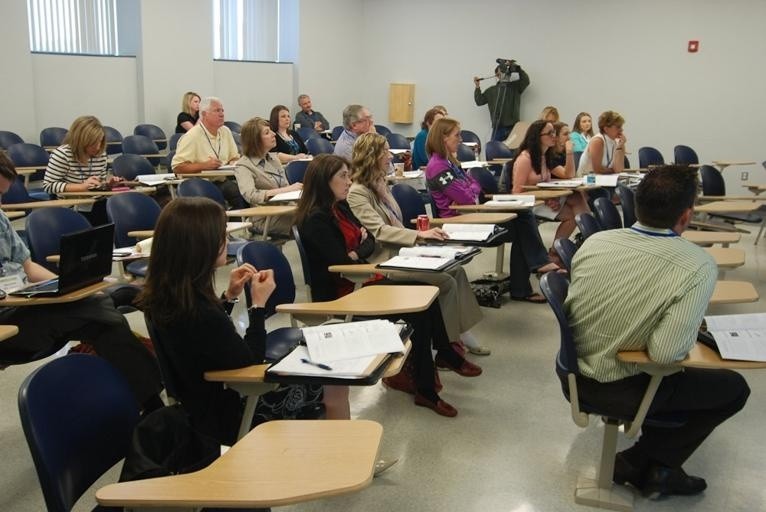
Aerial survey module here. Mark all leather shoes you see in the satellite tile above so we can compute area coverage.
[461,341,491,355]
[374,458,398,476]
[613,451,707,493]
[435,353,482,376]
[415,392,457,417]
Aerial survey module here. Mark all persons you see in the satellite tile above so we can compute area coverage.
[512,106,627,263]
[474,64,530,143]
[42,116,123,223]
[562,164,751,498]
[141,197,399,475]
[297,105,565,418]
[0,155,59,301]
[176,91,330,238]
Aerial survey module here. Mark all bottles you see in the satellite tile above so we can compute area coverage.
[474,144,479,162]
[587,171,595,185]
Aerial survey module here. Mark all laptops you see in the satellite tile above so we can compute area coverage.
[8,223,115,296]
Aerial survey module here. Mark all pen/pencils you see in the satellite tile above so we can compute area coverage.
[300,359,331,370]
[420,255,445,258]
[498,200,517,202]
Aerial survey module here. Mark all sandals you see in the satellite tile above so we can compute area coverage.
[511,293,547,302]
[532,267,568,273]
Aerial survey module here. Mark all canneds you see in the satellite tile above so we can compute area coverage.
[417,215,429,231]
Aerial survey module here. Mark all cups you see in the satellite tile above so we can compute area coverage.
[294,123,301,131]
[393,162,405,177]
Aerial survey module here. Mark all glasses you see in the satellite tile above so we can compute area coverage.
[540,129,556,136]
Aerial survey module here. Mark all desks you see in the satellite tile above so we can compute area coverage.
[616,333,766,439]
[200,340,412,441]
[94,417,383,510]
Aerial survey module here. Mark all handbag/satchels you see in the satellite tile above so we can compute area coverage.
[469,279,502,307]
[119,403,220,479]
[383,350,442,396]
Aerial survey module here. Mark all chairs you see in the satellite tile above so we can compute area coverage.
[536,274,656,506]
[18,353,138,510]
[0,112,764,365]
